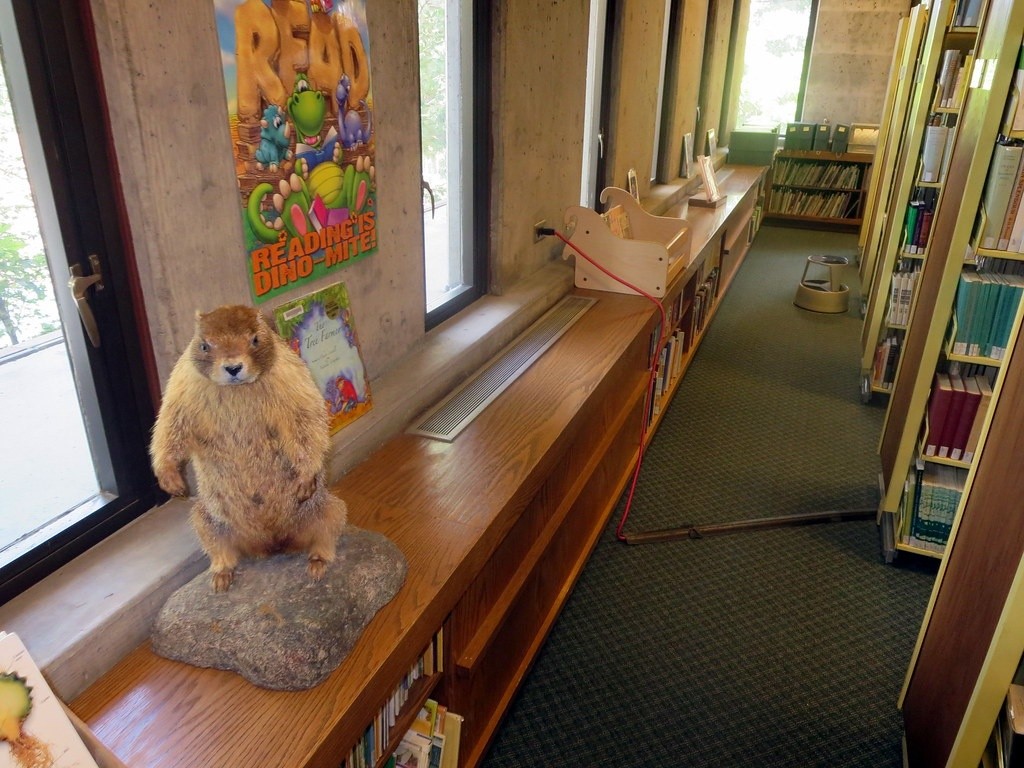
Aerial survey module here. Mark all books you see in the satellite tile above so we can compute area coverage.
[699,153,722,204]
[867,0,1024,553]
[1000,685,1024,767]
[628,170,641,206]
[683,132,696,178]
[634,257,723,434]
[743,174,770,244]
[598,204,636,242]
[271,281,376,437]
[706,128,718,160]
[0,630,119,768]
[327,618,469,768]
[767,157,870,220]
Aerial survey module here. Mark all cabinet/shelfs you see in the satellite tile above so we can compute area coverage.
[762,142,873,232]
[874,1,1023,564]
[896,291,1024,768]
[64,163,772,768]
[859,0,989,402]
